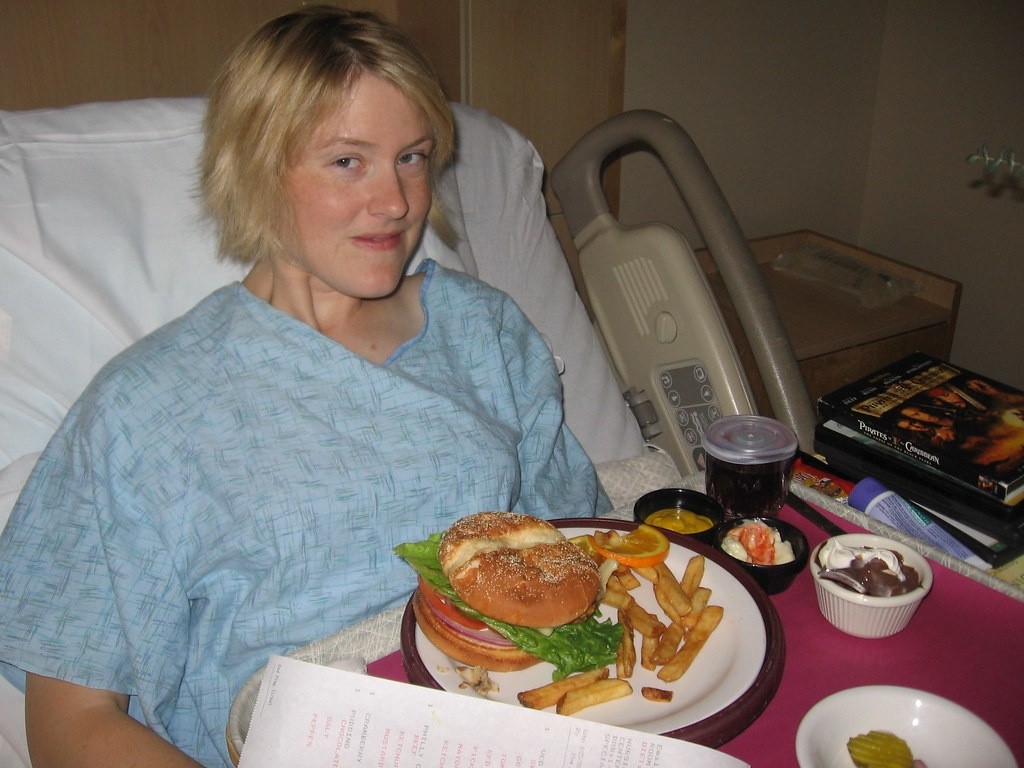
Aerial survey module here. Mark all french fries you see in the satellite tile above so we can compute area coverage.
[518,555,724,715]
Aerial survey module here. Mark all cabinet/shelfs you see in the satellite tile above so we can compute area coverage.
[689,228,965,417]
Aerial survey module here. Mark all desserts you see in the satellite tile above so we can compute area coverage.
[815,541,917,596]
[721,519,794,565]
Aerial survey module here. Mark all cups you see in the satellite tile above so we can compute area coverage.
[704,414,797,524]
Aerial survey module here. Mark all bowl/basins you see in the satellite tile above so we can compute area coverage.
[811,535,933,639]
[720,510,809,594]
[635,488,722,546]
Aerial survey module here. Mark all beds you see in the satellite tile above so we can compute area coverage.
[1,94,818,768]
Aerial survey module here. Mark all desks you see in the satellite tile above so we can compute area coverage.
[227,472,1024,768]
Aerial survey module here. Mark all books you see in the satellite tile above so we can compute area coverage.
[815,352,1024,569]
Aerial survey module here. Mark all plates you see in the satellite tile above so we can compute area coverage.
[416,526,766,735]
[795,686,1021,768]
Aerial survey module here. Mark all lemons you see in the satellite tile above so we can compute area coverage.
[587,524,669,566]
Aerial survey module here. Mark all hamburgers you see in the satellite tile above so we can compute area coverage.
[391,506,623,681]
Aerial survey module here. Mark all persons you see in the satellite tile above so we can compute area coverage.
[0,6,617,768]
[891,380,969,441]
